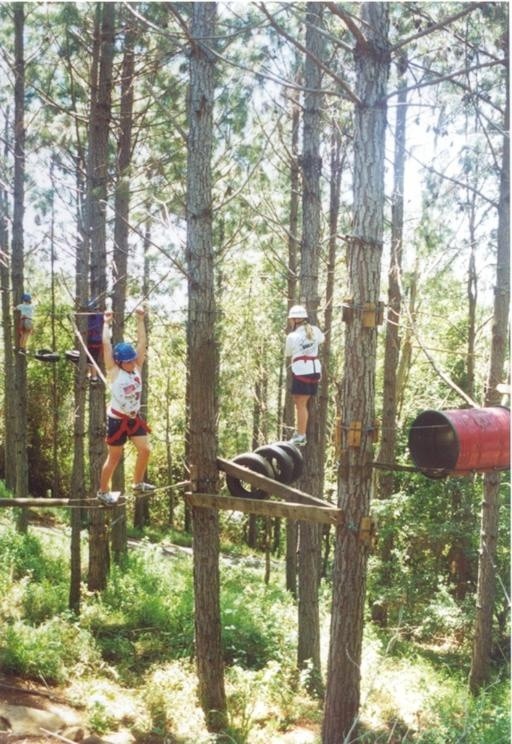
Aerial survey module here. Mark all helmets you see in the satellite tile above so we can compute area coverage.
[112,343,137,362]
[87,300,96,309]
[287,305,307,318]
[23,295,31,300]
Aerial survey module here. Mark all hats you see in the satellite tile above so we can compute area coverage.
[18,347,23,352]
[23,350,30,354]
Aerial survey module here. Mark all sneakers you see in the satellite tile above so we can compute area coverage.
[86,376,101,384]
[289,430,307,445]
[132,482,155,493]
[96,490,118,506]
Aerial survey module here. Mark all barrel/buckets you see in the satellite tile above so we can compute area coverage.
[406,404,510,480]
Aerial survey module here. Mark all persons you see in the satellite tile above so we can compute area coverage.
[15,293,36,355]
[283,303,327,445]
[98,305,156,506]
[85,297,105,385]
